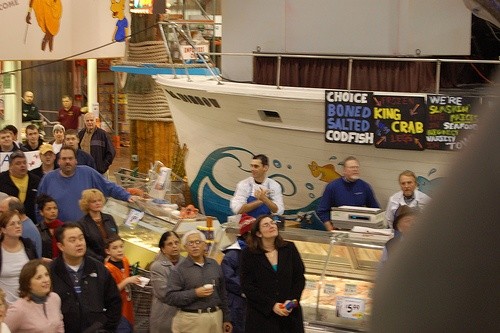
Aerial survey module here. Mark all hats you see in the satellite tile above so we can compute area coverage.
[239,212,256,235]
[40,144,54,155]
[392,205,418,230]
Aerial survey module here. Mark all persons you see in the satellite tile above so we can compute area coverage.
[384,169,433,229]
[48,222,124,333]
[238,214,307,333]
[103,234,143,333]
[0,124,140,304]
[149,231,187,333]
[0,287,11,333]
[48,124,65,154]
[57,94,88,131]
[164,229,233,333]
[3,259,65,333]
[21,124,47,152]
[22,91,41,122]
[230,154,285,219]
[77,112,116,181]
[367,74,500,333]
[375,204,420,292]
[316,156,382,233]
[220,212,258,333]
[54,129,95,170]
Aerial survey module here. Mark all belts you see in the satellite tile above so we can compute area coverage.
[181,307,216,313]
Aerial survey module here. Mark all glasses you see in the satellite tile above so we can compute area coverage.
[189,241,203,246]
[263,221,277,227]
